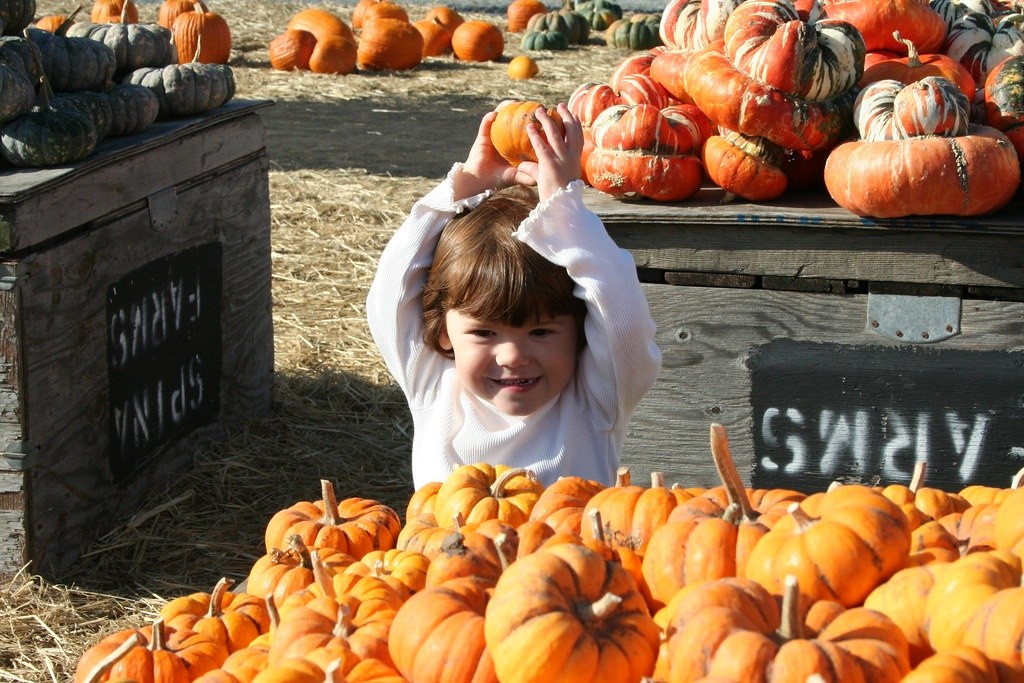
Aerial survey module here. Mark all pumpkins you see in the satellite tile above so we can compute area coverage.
[0,0,1024,218]
[75,421,1024,683]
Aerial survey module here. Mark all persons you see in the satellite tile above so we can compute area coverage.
[366,101,661,492]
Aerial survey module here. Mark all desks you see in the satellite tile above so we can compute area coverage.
[526,178,1024,496]
[0,97,279,579]
